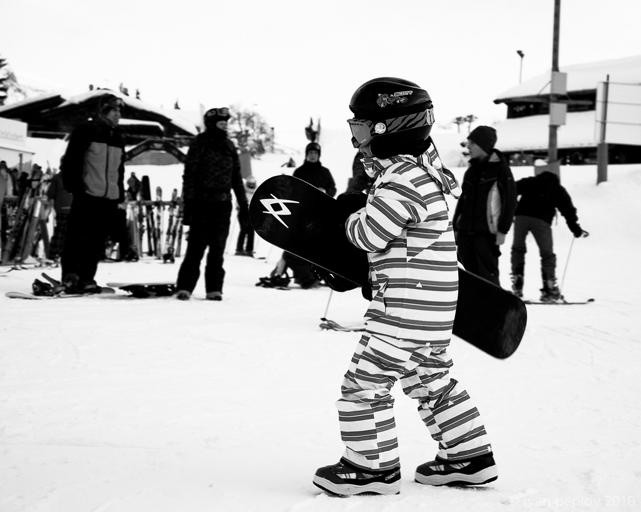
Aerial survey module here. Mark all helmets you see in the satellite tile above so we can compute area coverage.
[305,142,321,156]
[203,107,231,135]
[98,93,125,117]
[347,76,435,160]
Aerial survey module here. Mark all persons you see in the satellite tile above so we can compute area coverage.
[233,178,260,257]
[508,164,587,300]
[12,167,19,178]
[308,75,502,497]
[0,166,14,248]
[18,171,30,197]
[450,124,517,287]
[126,172,140,200]
[55,92,127,294]
[292,140,338,286]
[0,160,17,200]
[171,106,251,302]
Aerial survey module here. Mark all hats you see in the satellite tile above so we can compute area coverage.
[467,125,497,155]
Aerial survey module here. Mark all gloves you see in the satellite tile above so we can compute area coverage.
[334,190,367,219]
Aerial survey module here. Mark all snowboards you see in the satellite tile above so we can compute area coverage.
[0,161,183,267]
[5,292,139,299]
[318,318,366,332]
[524,299,594,304]
[249,176,526,360]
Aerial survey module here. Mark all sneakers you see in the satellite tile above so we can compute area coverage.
[414,449,499,487]
[177,290,191,300]
[312,457,401,497]
[204,291,222,301]
[539,288,564,302]
